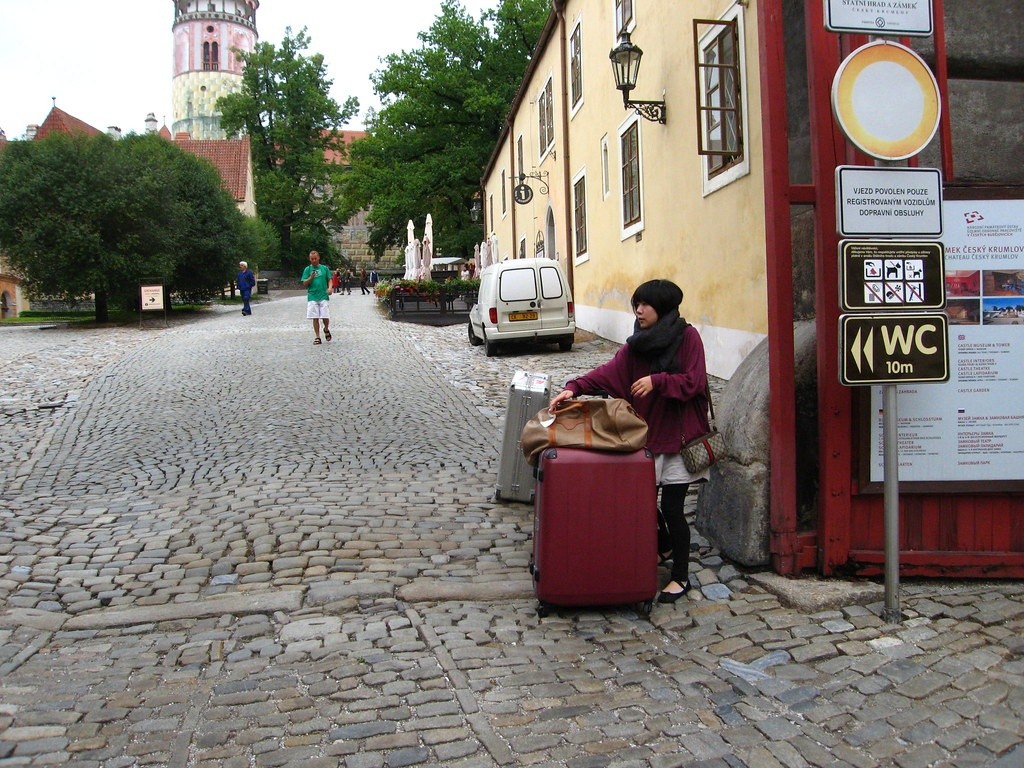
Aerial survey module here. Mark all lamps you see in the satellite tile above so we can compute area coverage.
[469,202,484,227]
[609,25,666,126]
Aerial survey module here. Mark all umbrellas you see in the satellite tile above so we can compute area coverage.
[474,233,499,278]
[404,213,434,282]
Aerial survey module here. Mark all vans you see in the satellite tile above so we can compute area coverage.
[468,258,577,357]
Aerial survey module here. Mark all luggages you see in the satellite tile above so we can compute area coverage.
[495,370,552,505]
[528,389,658,615]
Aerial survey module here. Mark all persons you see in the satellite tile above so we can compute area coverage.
[237,261,256,316]
[300,251,332,345]
[549,280,710,603]
[460,266,470,280]
[360,268,370,295]
[333,267,352,295]
[370,268,380,294]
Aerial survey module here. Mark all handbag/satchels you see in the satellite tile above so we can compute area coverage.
[682,431,727,473]
[520,399,648,468]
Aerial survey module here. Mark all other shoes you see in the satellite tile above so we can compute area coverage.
[657,552,673,566]
[242,311,246,316]
[658,579,691,603]
[246,313,251,315]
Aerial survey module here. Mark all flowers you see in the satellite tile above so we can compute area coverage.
[374,275,480,308]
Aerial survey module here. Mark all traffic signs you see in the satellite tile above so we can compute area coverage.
[837,315,951,386]
[141,286,164,310]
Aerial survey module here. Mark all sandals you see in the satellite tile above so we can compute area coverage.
[313,338,321,344]
[323,328,331,341]
